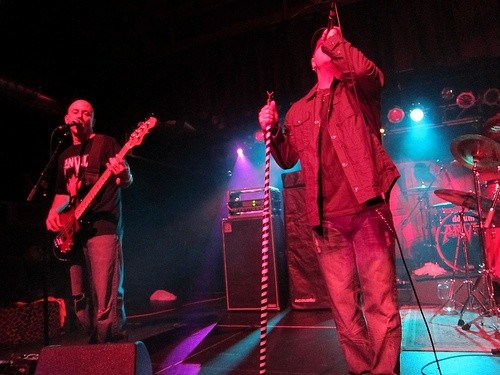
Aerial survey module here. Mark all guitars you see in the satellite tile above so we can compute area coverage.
[54,116,157,255]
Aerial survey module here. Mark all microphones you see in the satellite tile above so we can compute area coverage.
[58,120,82,130]
[438,161,450,182]
[325,3,336,41]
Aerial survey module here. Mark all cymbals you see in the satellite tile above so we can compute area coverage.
[405,186,444,191]
[430,201,456,208]
[449,134,500,170]
[434,188,500,214]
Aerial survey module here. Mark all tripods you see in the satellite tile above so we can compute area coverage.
[427,155,500,332]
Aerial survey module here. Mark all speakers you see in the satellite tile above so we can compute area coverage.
[35,341,153,375]
[221,214,289,311]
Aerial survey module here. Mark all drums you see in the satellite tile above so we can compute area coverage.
[475,179,500,202]
[480,225,500,283]
[435,211,487,271]
[433,212,452,224]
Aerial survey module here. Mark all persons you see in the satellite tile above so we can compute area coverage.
[258,25,401,375]
[46,100,133,344]
[484,165,500,318]
